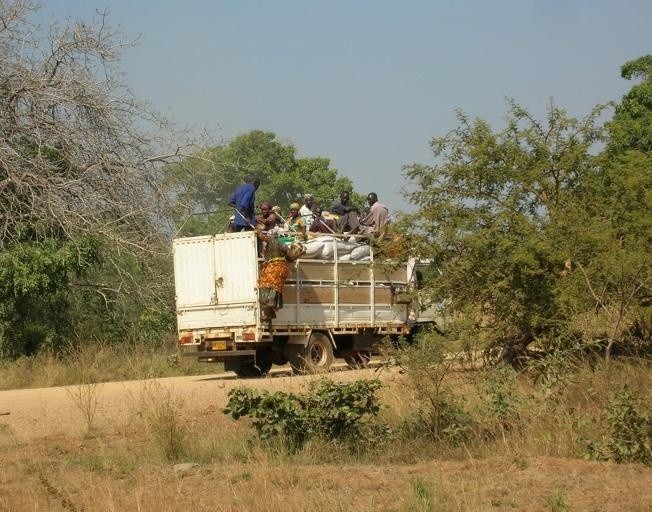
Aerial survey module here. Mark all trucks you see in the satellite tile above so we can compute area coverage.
[172,226,448,376]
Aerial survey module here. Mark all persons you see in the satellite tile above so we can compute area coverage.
[255,227,308,323]
[226,190,378,247]
[227,177,260,232]
[359,192,389,239]
